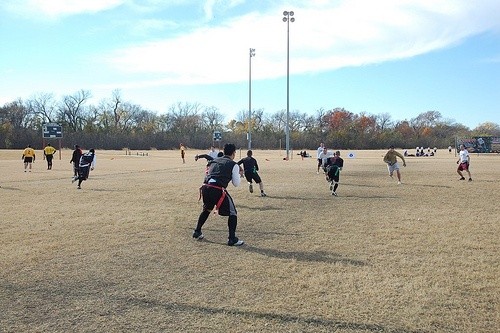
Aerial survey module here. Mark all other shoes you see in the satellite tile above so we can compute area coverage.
[390,176,394,180]
[77,186,81,189]
[198,234,204,238]
[332,193,338,196]
[469,178,472,182]
[72,179,74,183]
[330,186,332,190]
[460,177,465,180]
[261,193,267,197]
[249,185,253,193]
[398,181,401,184]
[234,241,244,245]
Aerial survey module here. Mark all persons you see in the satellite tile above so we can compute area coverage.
[193,143,244,246]
[382,147,406,184]
[180,143,185,163]
[22,144,36,172]
[317,143,325,174]
[456,144,472,182]
[324,151,344,196]
[72,148,96,189]
[70,144,82,177]
[44,143,56,170]
[319,148,334,168]
[237,150,266,196]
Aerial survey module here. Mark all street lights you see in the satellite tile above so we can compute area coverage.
[282,10,295,158]
[248,47,256,150]
